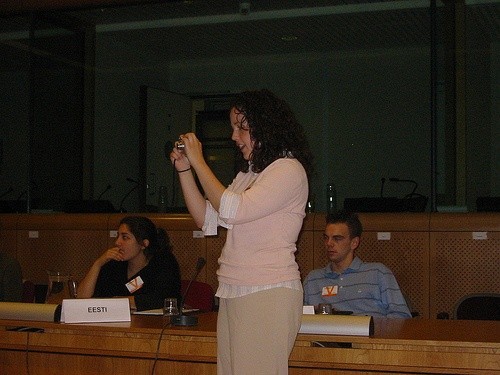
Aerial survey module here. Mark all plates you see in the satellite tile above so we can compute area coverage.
[133,308,199,315]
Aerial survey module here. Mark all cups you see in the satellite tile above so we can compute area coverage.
[163,299,178,320]
[318,304,332,315]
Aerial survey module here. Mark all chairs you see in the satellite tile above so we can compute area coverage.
[453,292,500,320]
[181,280,213,312]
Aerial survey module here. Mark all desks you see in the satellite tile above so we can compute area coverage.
[0,312,500,375]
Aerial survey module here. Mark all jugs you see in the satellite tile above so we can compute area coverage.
[45,270,77,305]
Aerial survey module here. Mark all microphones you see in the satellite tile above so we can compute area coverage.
[0,188,15,199]
[171,257,205,326]
[99,185,111,200]
[380,177,385,198]
[112,178,139,214]
[388,178,417,198]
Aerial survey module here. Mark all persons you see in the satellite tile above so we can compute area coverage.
[74,216,180,313]
[170,89,308,375]
[302,212,411,319]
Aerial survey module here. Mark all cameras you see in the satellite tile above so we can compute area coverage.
[177,138,185,150]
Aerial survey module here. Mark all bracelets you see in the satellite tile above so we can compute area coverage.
[177,167,192,173]
